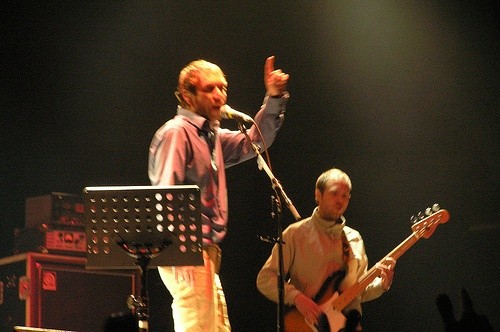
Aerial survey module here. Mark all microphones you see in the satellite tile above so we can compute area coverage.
[220,105,253,122]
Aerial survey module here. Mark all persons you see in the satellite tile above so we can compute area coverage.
[318,288,495,332]
[147,54,290,332]
[256,168,397,332]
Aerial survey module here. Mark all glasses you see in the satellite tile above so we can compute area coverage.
[174,90,185,104]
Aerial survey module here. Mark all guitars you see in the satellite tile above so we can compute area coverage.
[283,203,451,332]
